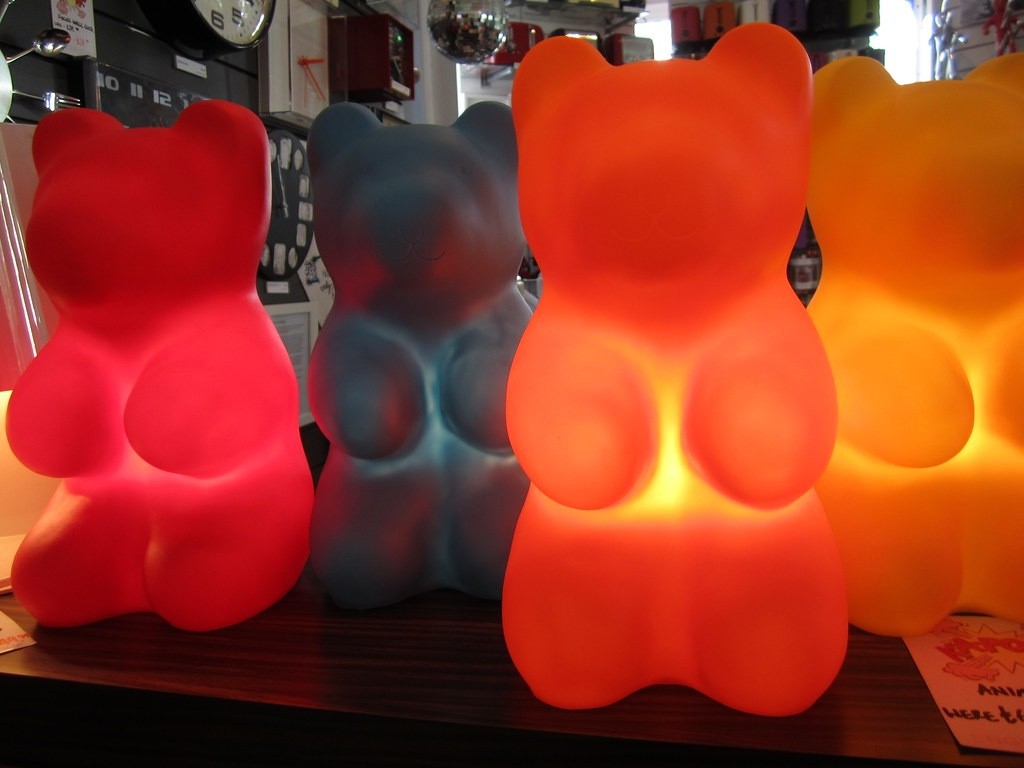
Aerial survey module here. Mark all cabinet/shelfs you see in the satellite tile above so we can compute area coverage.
[327,14,415,105]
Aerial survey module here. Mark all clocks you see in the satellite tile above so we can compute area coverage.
[138,0,276,60]
[258,130,313,281]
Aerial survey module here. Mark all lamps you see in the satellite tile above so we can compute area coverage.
[426,0,509,67]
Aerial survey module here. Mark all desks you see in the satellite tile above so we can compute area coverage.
[0,423,1024,768]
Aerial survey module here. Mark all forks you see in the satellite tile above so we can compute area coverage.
[13,89,82,112]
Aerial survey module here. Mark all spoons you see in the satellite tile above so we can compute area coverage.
[6,27,72,65]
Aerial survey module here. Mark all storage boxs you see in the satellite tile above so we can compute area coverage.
[258,0,347,138]
